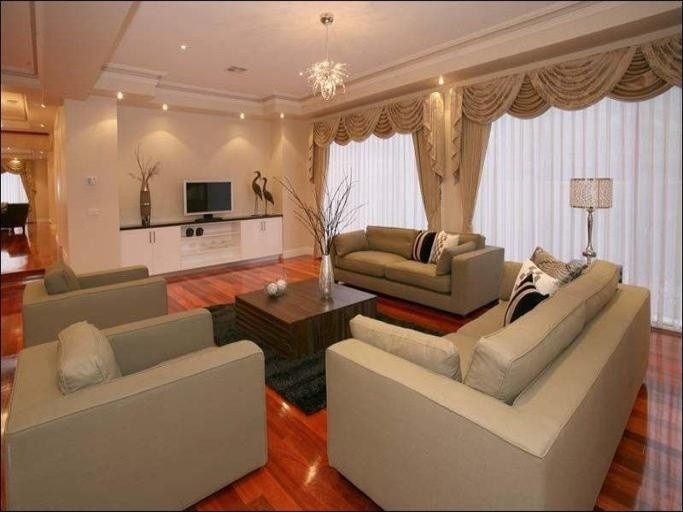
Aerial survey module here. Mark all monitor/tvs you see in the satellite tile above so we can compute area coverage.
[183,180,233,223]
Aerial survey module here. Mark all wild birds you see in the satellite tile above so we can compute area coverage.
[251,170,263,213]
[260,176,275,215]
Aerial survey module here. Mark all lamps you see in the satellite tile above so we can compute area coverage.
[299,14,352,104]
[569,177,613,268]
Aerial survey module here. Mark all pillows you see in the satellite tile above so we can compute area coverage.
[431,229,459,263]
[410,229,436,263]
[530,245,576,287]
[508,257,560,305]
[503,273,549,328]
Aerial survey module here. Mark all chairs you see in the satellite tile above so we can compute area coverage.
[1,307,268,512]
[21,265,169,349]
[0,201,31,236]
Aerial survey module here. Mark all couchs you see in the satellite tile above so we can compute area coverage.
[326,225,505,319]
[323,257,653,511]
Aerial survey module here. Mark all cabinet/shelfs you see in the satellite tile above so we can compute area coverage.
[239,218,282,263]
[119,226,180,276]
[181,222,240,270]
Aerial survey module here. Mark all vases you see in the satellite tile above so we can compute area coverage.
[139,181,151,225]
[318,254,335,300]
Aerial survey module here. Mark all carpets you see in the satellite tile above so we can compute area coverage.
[202,301,453,417]
